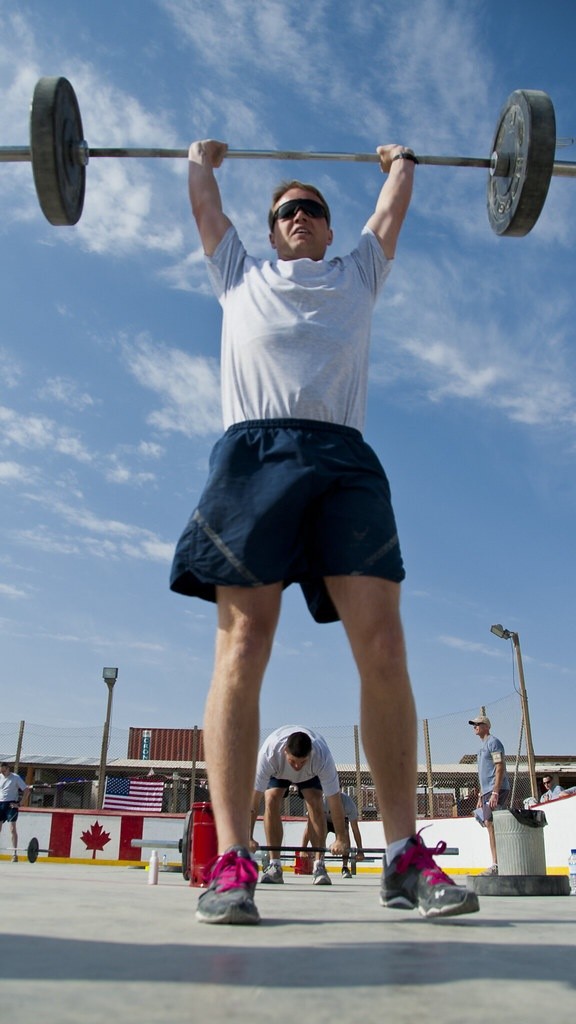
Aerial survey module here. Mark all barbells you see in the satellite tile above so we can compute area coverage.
[130,801,460,889]
[280,848,376,874]
[0,837,54,864]
[0,75,576,237]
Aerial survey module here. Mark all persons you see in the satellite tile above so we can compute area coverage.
[168,141,480,925]
[560,786,576,797]
[539,775,563,803]
[468,716,511,875]
[248,723,348,884]
[0,762,30,863]
[299,782,364,878]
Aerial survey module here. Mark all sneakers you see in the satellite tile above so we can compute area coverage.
[311,865,332,884]
[379,824,479,918]
[482,863,498,876]
[195,844,260,925]
[261,863,284,884]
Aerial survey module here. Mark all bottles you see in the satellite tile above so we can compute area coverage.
[261,849,270,873]
[148,850,159,885]
[569,849,576,896]
[162,854,167,867]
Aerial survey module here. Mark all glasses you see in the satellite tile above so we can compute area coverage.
[271,197,330,234]
[543,779,552,785]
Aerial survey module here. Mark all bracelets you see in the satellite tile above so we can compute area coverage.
[393,152,418,165]
[491,791,499,796]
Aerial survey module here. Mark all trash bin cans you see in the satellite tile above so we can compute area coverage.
[490,807,547,876]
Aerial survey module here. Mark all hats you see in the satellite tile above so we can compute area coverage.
[469,715,492,729]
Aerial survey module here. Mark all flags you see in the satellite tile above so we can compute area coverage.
[101,776,164,814]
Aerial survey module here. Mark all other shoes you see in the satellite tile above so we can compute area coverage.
[12,857,18,862]
[341,866,352,878]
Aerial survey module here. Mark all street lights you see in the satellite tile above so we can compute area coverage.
[96,666,119,808]
[491,624,540,803]
